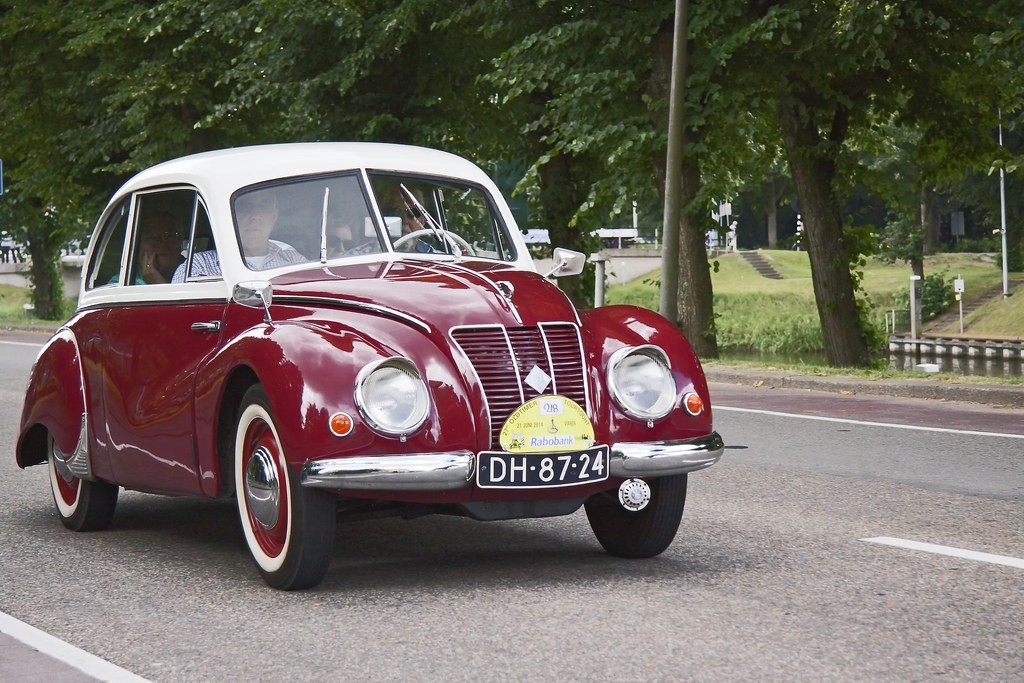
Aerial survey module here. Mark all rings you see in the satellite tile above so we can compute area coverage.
[146,264,150,268]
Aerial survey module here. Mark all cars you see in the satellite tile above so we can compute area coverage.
[15,141,723,594]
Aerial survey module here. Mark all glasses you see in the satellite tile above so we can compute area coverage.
[385,207,421,219]
[141,231,175,242]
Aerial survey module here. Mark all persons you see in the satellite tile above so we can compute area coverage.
[171,188,309,284]
[107,209,187,284]
[386,185,443,253]
